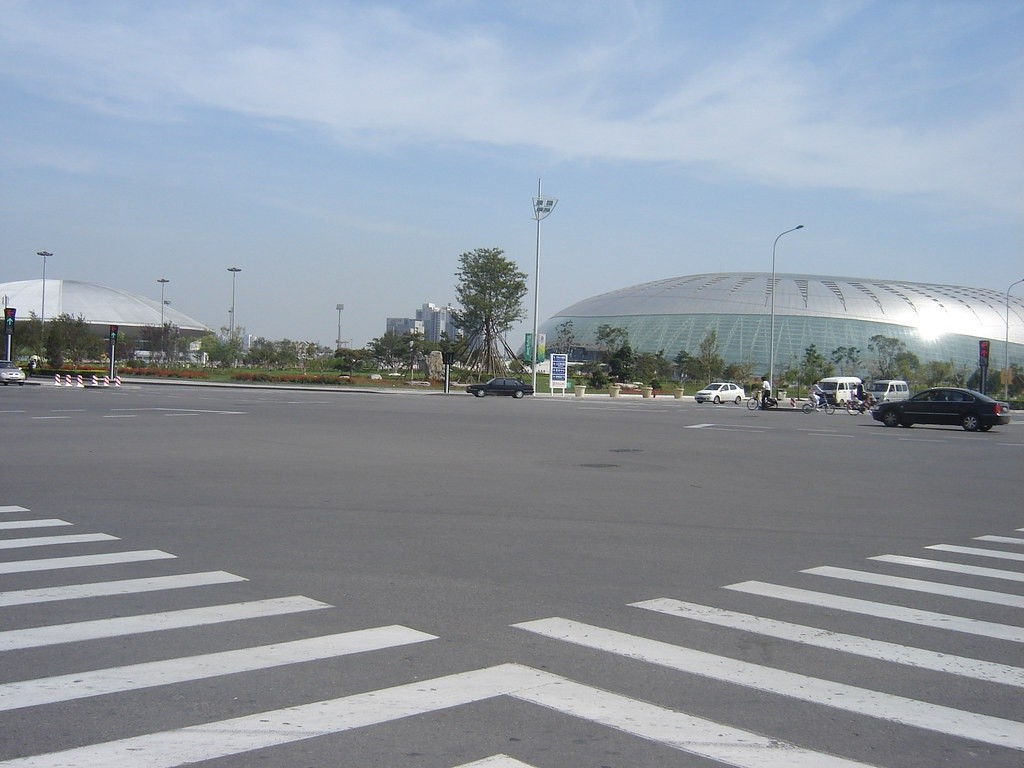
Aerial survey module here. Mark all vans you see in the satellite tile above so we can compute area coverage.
[813,376,862,409]
[865,380,911,408]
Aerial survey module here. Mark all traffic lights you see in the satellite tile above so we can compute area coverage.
[109,325,118,345]
[979,340,991,366]
[4,308,17,336]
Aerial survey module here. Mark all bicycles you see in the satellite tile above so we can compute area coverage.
[846,393,880,416]
[747,388,779,411]
[802,391,836,415]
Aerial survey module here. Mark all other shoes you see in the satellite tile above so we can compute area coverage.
[816,408,820,411]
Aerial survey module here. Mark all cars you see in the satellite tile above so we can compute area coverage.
[466,377,535,399]
[694,382,746,404]
[872,387,1011,432]
[0,360,25,386]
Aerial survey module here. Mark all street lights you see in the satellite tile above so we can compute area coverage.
[1004,278,1024,402]
[36,251,54,366]
[157,278,170,351]
[336,303,344,349]
[769,224,805,397]
[227,267,242,340]
[531,177,559,396]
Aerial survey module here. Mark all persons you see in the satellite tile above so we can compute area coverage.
[851,380,868,414]
[809,380,826,411]
[758,376,770,409]
[28,359,37,376]
[649,379,656,398]
